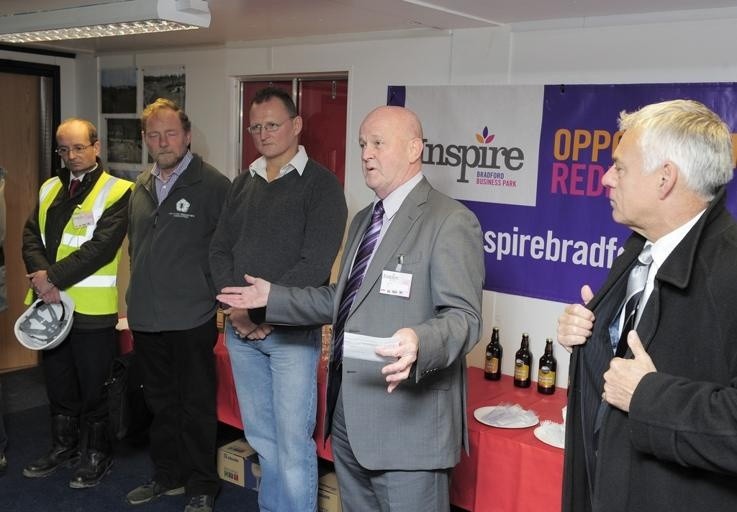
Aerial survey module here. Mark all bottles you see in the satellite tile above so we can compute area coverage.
[514,333,533,389]
[538,339,557,396]
[484,327,503,381]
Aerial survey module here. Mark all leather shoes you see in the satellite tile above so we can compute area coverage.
[68,448,114,490]
[22,445,83,478]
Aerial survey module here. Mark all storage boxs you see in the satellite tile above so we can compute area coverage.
[217,438,341,511]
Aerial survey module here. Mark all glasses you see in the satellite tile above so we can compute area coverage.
[56,143,92,155]
[247,115,293,132]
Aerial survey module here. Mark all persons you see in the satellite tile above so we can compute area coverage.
[125,97,232,511]
[214,106,486,510]
[208,90,348,512]
[12,122,136,490]
[557,99,737,512]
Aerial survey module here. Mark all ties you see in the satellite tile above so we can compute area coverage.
[616,245,653,361]
[332,201,384,370]
[69,179,81,196]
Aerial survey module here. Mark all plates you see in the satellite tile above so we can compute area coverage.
[473,406,540,429]
[533,424,565,449]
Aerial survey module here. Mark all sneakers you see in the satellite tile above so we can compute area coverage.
[126,478,186,505]
[183,491,217,512]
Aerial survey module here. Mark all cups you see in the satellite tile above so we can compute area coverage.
[250,463,264,492]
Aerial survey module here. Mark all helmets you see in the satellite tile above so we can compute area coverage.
[14,292,76,352]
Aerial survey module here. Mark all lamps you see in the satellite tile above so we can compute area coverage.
[0,0,212,43]
